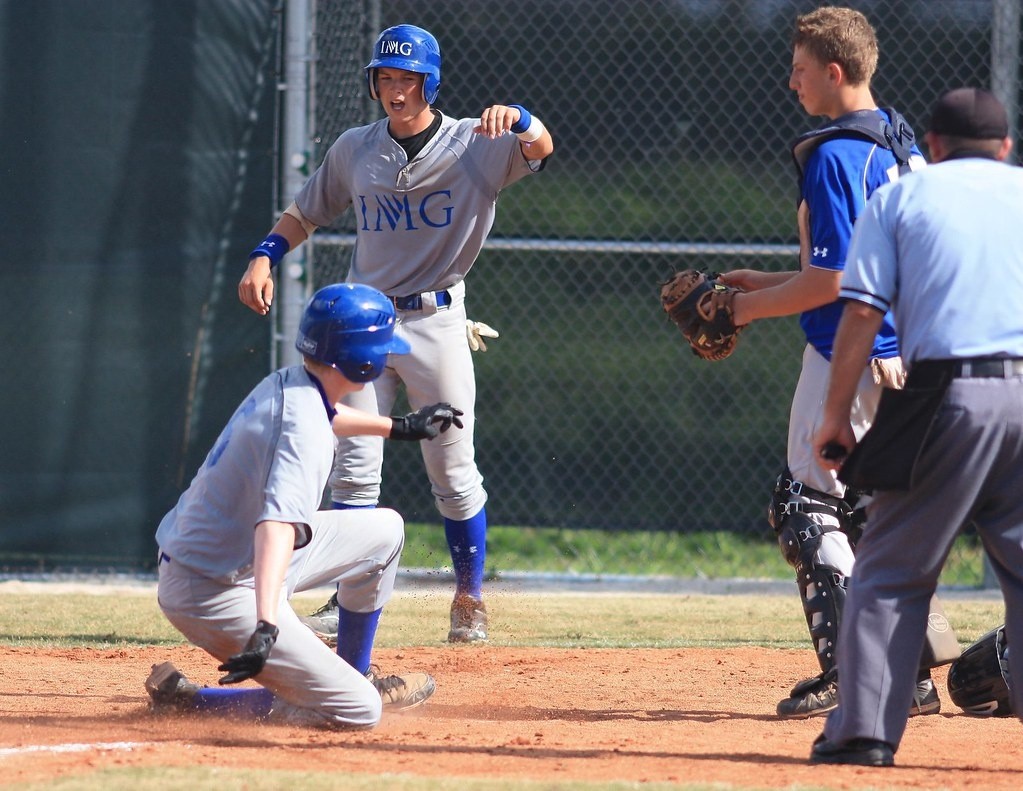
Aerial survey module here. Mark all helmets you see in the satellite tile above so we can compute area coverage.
[947,624,1013,717]
[292,282,411,384]
[363,23,441,104]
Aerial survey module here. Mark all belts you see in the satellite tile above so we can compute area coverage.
[954,356,1023,380]
[386,289,451,312]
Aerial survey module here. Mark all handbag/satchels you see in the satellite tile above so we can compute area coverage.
[836,367,955,493]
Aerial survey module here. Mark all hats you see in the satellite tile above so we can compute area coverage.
[929,87,1009,140]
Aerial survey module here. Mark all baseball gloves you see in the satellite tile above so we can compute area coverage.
[660,271,748,364]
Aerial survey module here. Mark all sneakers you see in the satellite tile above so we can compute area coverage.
[144,660,200,720]
[909,679,941,718]
[772,667,840,720]
[295,592,340,647]
[446,591,487,646]
[364,663,436,715]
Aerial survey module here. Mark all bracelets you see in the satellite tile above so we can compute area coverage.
[507,104,543,143]
[250,233,290,268]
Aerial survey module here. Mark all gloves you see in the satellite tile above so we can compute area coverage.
[217,619,281,685]
[389,401,464,442]
[466,319,500,353]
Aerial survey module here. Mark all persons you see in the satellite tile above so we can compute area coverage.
[661,6,942,717]
[238,26,555,642]
[145,282,464,732]
[810,88,1023,767]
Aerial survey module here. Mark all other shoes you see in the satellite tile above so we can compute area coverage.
[807,732,896,768]
